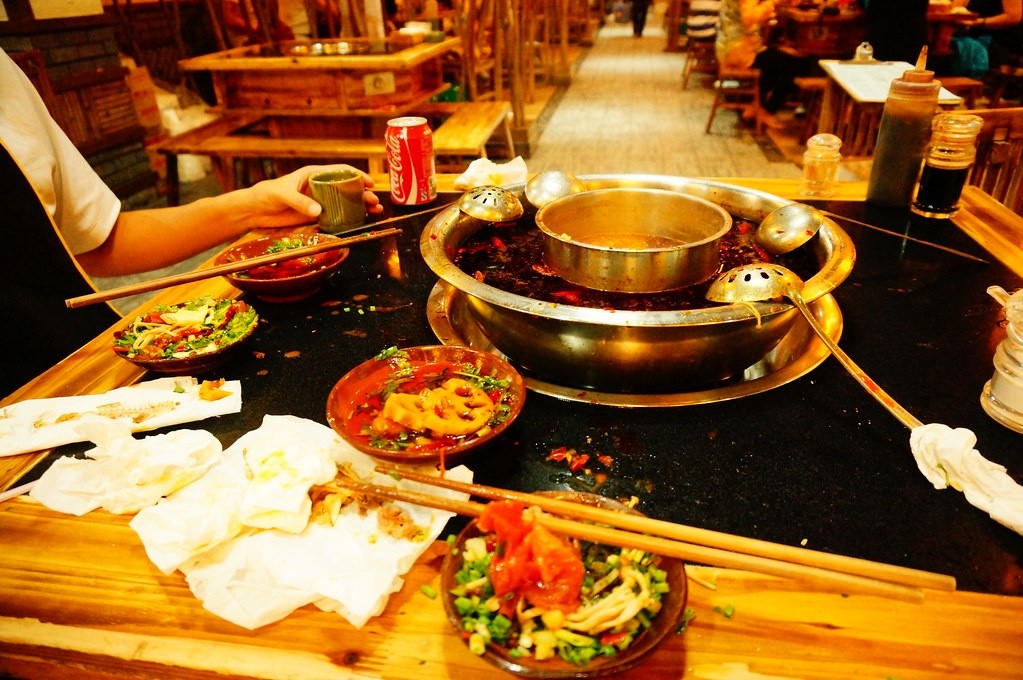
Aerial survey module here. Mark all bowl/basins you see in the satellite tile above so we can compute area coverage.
[213,233,350,302]
[324,343,527,466]
[111,298,262,375]
[441,491,688,680]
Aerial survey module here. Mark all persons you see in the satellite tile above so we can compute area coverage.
[622,0,656,38]
[686,0,1023,130]
[0,47,383,402]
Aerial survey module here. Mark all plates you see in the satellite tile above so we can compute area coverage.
[0,380,242,459]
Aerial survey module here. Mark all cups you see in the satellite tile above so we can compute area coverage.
[306,169,370,234]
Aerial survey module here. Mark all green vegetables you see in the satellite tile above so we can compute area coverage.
[233,239,327,279]
[112,294,260,359]
[356,345,513,451]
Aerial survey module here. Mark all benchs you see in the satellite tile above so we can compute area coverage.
[150,32,1023,216]
[794,77,983,147]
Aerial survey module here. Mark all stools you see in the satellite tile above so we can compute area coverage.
[704,66,765,139]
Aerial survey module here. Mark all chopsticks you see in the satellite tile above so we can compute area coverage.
[65,226,405,308]
[336,465,957,606]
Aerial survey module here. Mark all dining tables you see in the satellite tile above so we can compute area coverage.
[0,172,1023,680]
[174,34,461,143]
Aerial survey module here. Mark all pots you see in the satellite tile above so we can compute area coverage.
[420,171,859,396]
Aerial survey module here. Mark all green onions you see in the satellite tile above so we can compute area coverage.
[417,534,732,666]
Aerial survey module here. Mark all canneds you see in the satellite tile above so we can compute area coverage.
[385,116,438,205]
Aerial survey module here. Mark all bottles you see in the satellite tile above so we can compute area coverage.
[910,113,983,220]
[979,285,1023,434]
[799,132,843,198]
[866,45,942,221]
